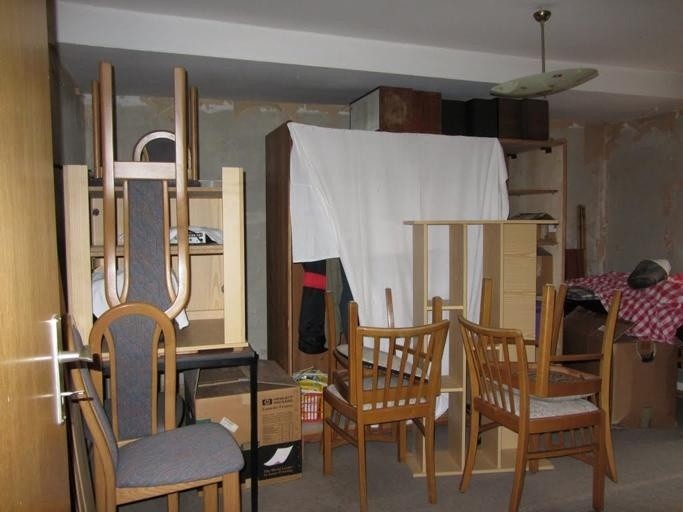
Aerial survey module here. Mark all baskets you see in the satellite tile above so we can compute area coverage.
[300,393,322,422]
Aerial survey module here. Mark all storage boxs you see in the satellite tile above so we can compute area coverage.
[183,360,303,496]
[562,308,679,431]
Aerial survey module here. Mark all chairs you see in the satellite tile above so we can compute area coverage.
[64,314,244,511]
[456,314,607,512]
[87,303,187,510]
[322,287,443,450]
[473,277,622,483]
[87,60,201,317]
[322,321,450,511]
[133,131,192,178]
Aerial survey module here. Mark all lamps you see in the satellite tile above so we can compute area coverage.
[490,10,598,100]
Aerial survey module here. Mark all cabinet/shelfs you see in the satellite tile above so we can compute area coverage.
[497,137,569,364]
[402,218,558,477]
[60,164,249,352]
[266,121,453,443]
